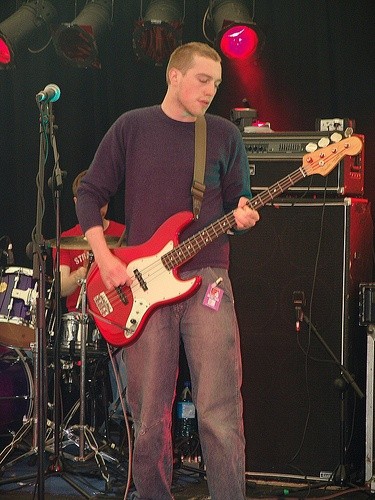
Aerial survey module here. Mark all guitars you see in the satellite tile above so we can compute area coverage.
[86,124,363,349]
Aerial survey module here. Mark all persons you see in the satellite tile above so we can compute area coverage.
[52,170,126,313]
[76,42,260,500]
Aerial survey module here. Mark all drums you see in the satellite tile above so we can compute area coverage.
[0,264,59,351]
[50,310,108,362]
[0,347,37,451]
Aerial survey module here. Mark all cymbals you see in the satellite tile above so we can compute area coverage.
[42,234,128,251]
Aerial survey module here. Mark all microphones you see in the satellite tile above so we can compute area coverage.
[36,83,61,103]
[293,290,306,334]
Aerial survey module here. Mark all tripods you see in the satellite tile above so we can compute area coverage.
[0,101,127,500]
[276,313,375,498]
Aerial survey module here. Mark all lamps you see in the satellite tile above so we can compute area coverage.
[0,0,55,70]
[132,0,186,67]
[51,0,114,73]
[202,0,265,62]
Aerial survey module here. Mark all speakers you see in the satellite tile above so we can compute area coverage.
[228,197,354,484]
